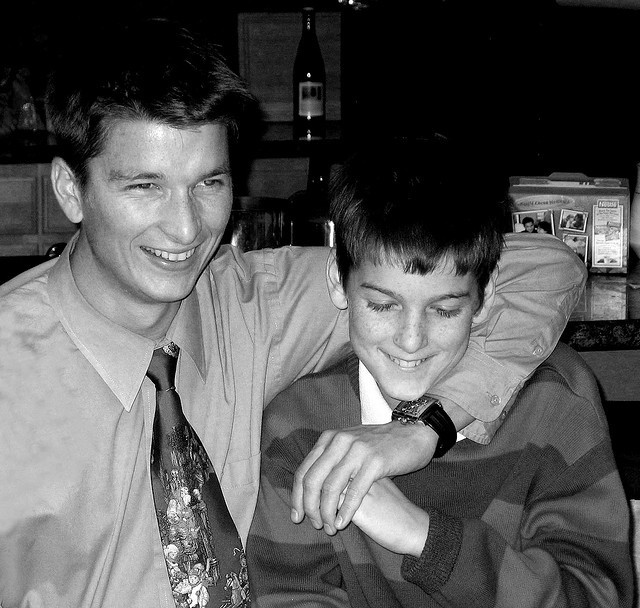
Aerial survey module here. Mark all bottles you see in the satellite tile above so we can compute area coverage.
[290,7,326,140]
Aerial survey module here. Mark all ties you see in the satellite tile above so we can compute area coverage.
[145,342,251,608]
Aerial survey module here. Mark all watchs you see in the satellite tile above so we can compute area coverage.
[390,394,458,458]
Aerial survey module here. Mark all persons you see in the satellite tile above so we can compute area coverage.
[538,222,550,233]
[244,115,638,605]
[569,210,586,232]
[518,217,537,233]
[0,11,589,607]
